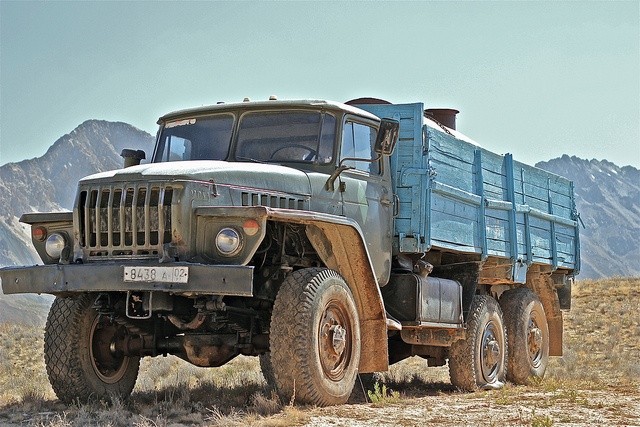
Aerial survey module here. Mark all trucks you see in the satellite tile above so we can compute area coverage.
[0,94,585,410]
